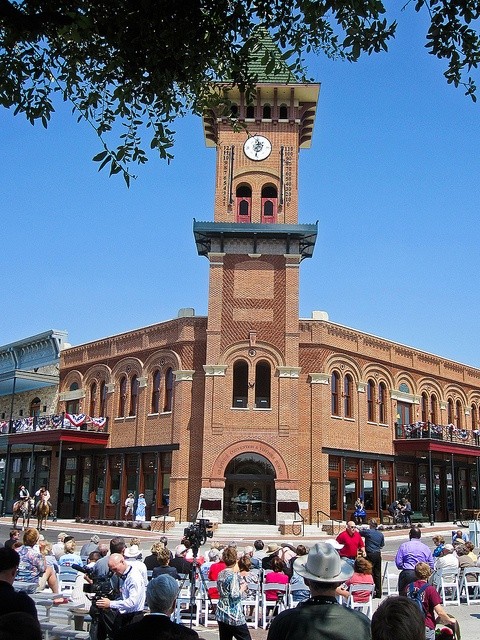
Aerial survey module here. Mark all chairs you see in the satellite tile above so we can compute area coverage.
[240,583,260,630]
[203,581,219,628]
[263,569,284,585]
[56,573,76,593]
[338,593,343,606]
[57,564,78,575]
[176,579,202,628]
[262,582,288,630]
[288,583,312,610]
[459,567,480,606]
[436,567,461,607]
[346,582,375,619]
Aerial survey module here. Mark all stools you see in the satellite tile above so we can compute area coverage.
[67,613,93,632]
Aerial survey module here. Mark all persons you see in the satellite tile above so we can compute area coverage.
[92,537,128,640]
[52,532,69,561]
[387,501,399,525]
[279,542,296,569]
[160,537,173,561]
[96,553,145,628]
[401,499,412,527]
[80,535,100,565]
[432,535,446,557]
[277,574,310,613]
[371,594,426,640]
[152,548,177,580]
[395,528,435,597]
[289,545,309,576]
[465,541,477,566]
[456,546,477,601]
[0,548,42,640]
[12,529,67,605]
[452,531,467,545]
[215,547,251,640]
[260,543,287,595]
[123,545,149,610]
[125,493,134,521]
[17,484,32,513]
[201,548,220,569]
[0,530,23,549]
[58,540,84,589]
[39,540,59,574]
[353,496,367,526]
[434,544,459,595]
[208,549,226,613]
[335,557,375,611]
[229,542,244,560]
[237,557,259,622]
[34,485,54,516]
[244,546,263,591]
[266,558,289,629]
[336,521,367,567]
[252,540,270,568]
[404,563,456,640]
[144,541,171,581]
[169,545,198,609]
[267,543,371,640]
[402,498,406,506]
[359,519,384,598]
[68,551,100,614]
[135,494,147,521]
[114,574,199,640]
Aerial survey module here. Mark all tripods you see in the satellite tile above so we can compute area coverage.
[178,549,215,629]
[265,591,287,630]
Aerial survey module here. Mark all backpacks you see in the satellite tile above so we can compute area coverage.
[407,582,432,620]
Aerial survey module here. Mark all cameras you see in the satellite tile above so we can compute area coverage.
[181,520,213,549]
[71,563,119,607]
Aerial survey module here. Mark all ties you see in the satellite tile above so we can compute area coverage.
[120,565,133,583]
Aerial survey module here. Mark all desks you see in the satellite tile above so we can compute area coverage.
[27,593,71,622]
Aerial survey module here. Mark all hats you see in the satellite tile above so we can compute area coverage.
[138,493,144,497]
[266,543,283,554]
[441,544,454,553]
[292,541,355,583]
[281,541,298,554]
[244,546,254,555]
[123,544,143,557]
[208,548,222,562]
[127,492,134,497]
